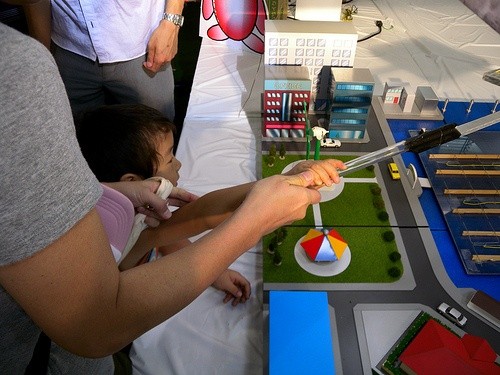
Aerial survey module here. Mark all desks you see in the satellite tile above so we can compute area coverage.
[129,0,500,375]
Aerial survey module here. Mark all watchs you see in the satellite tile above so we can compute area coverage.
[163,13,185,27]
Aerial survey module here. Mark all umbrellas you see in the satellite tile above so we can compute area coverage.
[300,227,346,262]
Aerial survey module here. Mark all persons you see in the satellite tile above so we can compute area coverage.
[0,23,322,375]
[80,105,347,305]
[22,0,186,123]
